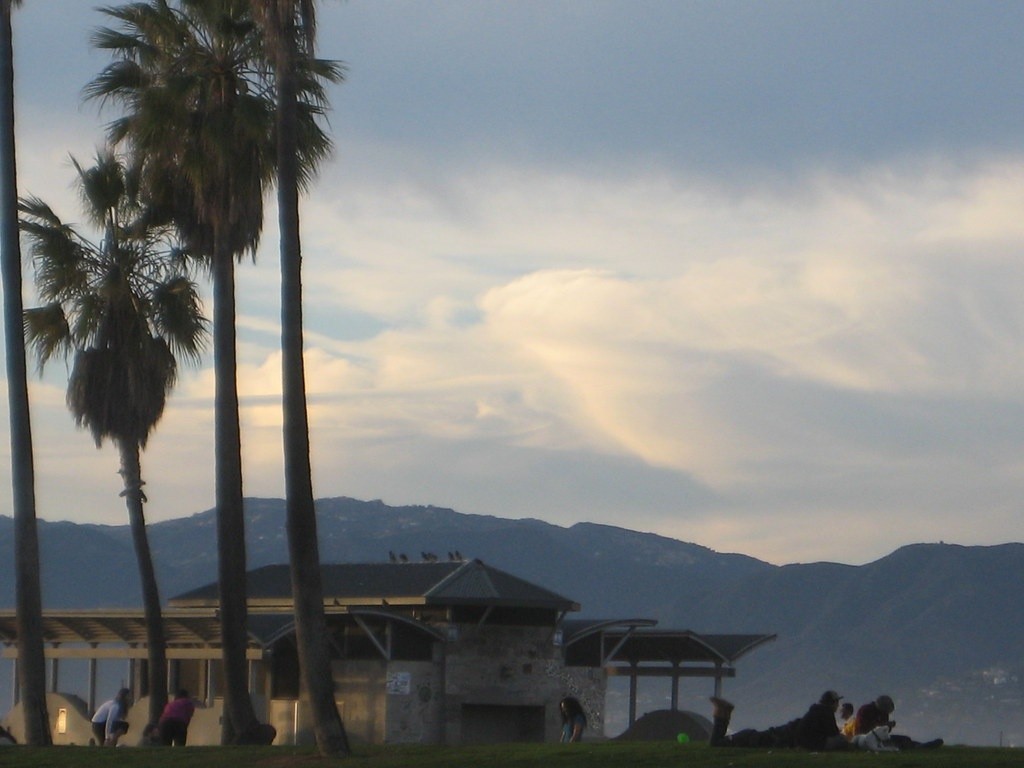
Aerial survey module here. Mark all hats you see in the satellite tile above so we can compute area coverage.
[820,690,844,703]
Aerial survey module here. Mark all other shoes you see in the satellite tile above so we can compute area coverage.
[709,695,735,719]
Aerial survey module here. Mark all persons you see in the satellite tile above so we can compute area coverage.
[707,690,848,748]
[856,695,944,750]
[560,696,586,742]
[90,686,134,747]
[839,703,856,742]
[157,692,195,745]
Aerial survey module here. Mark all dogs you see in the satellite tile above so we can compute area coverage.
[851,725,900,752]
